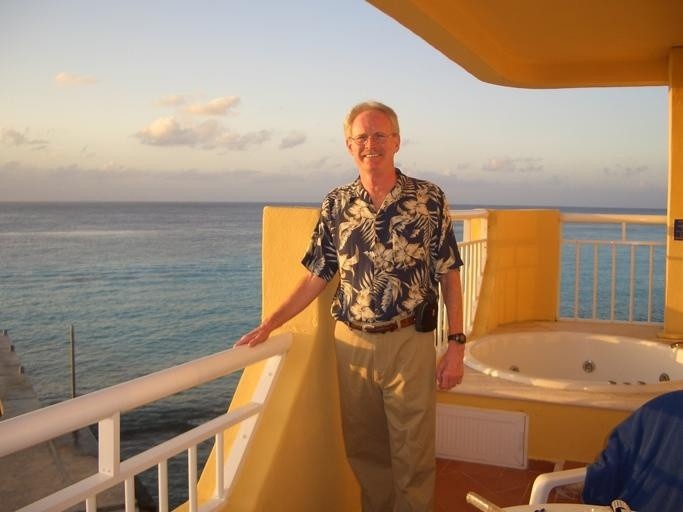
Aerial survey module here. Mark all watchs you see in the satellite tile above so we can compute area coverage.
[448,333,466,344]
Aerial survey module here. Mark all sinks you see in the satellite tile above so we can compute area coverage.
[464,330,683,390]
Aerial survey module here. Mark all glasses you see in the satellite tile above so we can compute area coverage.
[350,132,396,145]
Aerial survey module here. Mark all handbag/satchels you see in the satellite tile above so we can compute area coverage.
[415,300,437,331]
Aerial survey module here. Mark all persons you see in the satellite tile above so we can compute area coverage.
[234,100,466,511]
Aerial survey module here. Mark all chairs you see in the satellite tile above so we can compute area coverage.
[520,390,682,512]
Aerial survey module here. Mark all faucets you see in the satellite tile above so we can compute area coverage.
[669,340,682,349]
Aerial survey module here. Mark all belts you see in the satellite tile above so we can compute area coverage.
[344,315,415,333]
